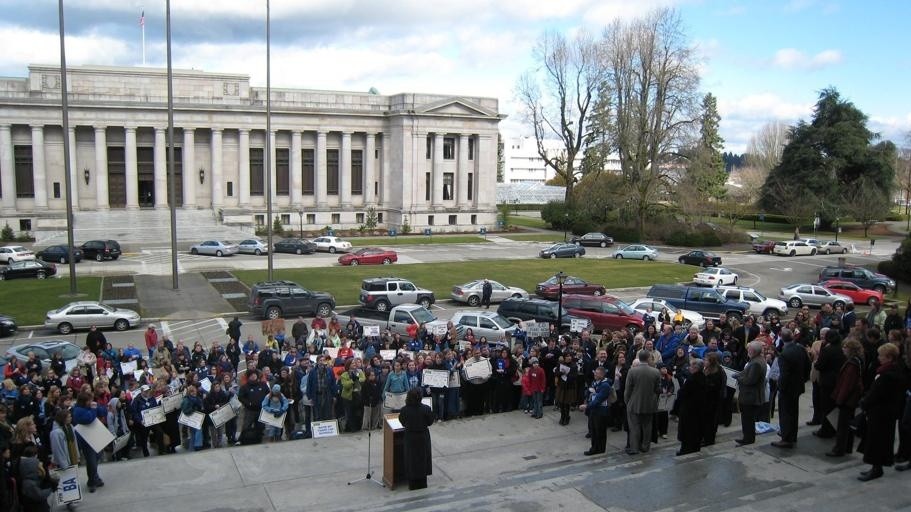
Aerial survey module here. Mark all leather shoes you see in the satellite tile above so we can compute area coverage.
[857,469,882,481]
[771,440,792,447]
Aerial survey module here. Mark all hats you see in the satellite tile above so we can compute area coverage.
[531,357,539,364]
[272,384,281,393]
[298,358,308,361]
[140,385,150,392]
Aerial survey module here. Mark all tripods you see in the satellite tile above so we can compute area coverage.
[348,404,386,487]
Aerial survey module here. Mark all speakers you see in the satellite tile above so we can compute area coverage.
[359,379,382,407]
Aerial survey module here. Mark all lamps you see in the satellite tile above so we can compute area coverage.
[83,166,91,186]
[198,167,206,185]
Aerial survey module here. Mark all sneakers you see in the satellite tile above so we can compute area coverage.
[662,434,668,438]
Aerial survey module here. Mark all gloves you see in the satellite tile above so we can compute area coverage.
[271,408,280,413]
[275,410,284,417]
[351,371,358,381]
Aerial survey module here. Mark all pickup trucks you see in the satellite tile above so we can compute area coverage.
[331,303,464,344]
[648,284,752,327]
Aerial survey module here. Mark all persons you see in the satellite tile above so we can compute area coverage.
[482,279,492,306]
[1,314,910,510]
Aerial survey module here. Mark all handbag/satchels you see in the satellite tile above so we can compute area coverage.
[608,385,618,403]
[755,421,776,433]
[836,372,863,401]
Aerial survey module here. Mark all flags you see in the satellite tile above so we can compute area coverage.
[141,11,145,25]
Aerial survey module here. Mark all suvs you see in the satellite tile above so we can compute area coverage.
[775,240,818,258]
[248,279,336,321]
[360,275,437,313]
[562,294,647,336]
[498,297,595,342]
[704,286,789,323]
[819,265,896,295]
[79,240,122,261]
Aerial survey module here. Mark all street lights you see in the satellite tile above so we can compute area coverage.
[815,215,821,239]
[514,198,521,215]
[564,213,569,240]
[555,271,569,340]
[836,216,840,242]
[605,204,609,216]
[299,210,304,237]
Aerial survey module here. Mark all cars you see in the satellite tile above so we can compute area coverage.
[692,267,740,288]
[817,240,848,254]
[0,246,35,264]
[238,238,275,256]
[752,240,776,254]
[613,244,659,261]
[540,242,586,259]
[189,240,240,257]
[778,283,854,311]
[618,297,707,335]
[817,280,884,306]
[572,232,614,248]
[0,260,57,280]
[45,300,141,335]
[37,245,85,263]
[339,246,399,267]
[0,315,18,337]
[536,275,606,300]
[3,339,85,382]
[800,239,818,246]
[452,278,531,307]
[894,193,911,208]
[0,356,20,406]
[273,238,317,254]
[451,310,520,345]
[308,236,353,254]
[680,250,723,268]
